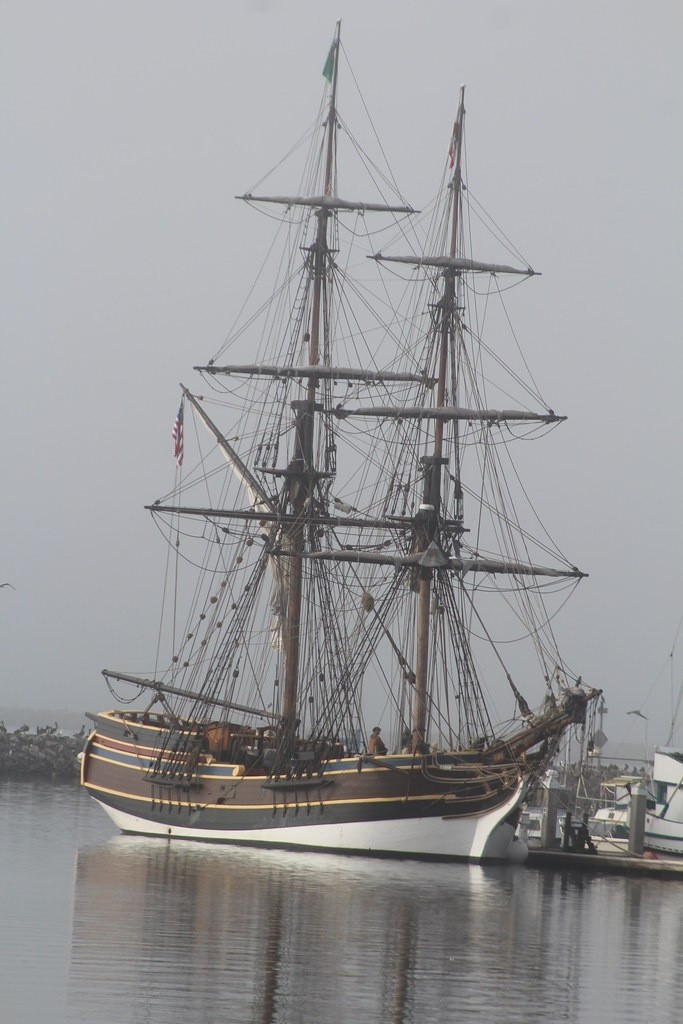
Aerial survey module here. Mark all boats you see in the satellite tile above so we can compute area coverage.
[513,676,682,871]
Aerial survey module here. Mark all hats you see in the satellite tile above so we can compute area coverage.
[373,727,381,731]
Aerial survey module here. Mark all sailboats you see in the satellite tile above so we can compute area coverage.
[71,14,611,862]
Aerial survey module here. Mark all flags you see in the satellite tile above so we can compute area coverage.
[171,401,183,467]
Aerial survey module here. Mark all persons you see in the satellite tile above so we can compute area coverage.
[369,727,388,755]
